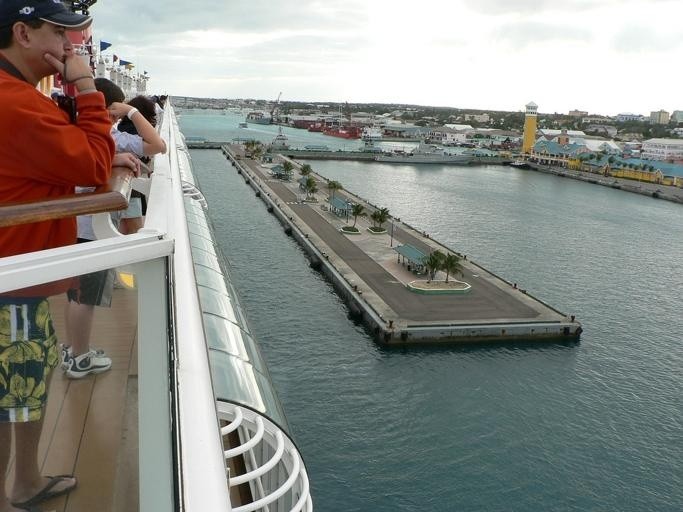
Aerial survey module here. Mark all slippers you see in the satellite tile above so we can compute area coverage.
[11,475,78,509]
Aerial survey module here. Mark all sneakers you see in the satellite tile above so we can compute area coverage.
[60,344,105,371]
[65,355,111,379]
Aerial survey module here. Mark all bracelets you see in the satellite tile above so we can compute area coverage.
[126,108,138,120]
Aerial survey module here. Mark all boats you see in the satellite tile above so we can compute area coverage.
[230,136,251,142]
[508,162,528,169]
[272,126,289,148]
[460,148,486,157]
[356,133,382,153]
[302,144,329,151]
[369,132,475,164]
[321,124,360,138]
[244,109,273,125]
[239,122,247,128]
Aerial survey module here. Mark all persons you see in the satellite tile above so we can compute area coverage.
[0,0,115,507]
[59,76,168,381]
[45,91,167,236]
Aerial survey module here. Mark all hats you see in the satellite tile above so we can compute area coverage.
[0,0,92,31]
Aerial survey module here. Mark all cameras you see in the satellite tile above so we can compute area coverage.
[58,94,76,126]
